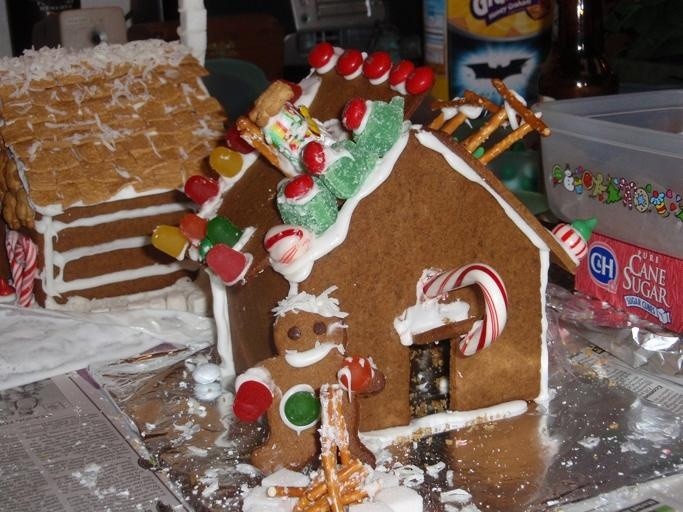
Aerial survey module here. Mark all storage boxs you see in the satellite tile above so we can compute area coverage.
[530,88,683,336]
